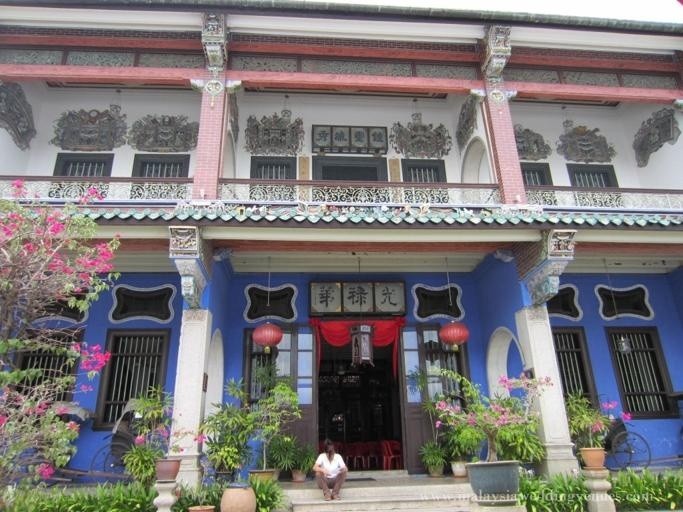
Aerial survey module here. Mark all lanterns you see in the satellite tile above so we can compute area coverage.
[348,323,376,369]
[250,322,283,354]
[437,319,469,351]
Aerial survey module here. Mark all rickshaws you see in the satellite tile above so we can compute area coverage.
[567,390,650,474]
[47,398,153,486]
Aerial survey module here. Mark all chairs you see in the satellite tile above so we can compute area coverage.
[319,439,402,470]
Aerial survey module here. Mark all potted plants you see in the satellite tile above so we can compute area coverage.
[405,364,467,477]
[567,388,611,467]
[188,358,316,512]
[496,417,548,478]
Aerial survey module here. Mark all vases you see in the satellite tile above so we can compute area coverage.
[154,457,182,482]
[465,461,520,495]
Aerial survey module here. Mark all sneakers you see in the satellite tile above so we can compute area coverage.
[324,494,341,501]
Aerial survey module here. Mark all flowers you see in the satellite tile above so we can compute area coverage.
[135,426,207,459]
[434,372,554,462]
[591,400,633,433]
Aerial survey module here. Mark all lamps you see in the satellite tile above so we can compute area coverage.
[349,255,375,369]
[601,258,632,354]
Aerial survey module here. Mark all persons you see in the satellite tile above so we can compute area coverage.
[311,438,348,501]
